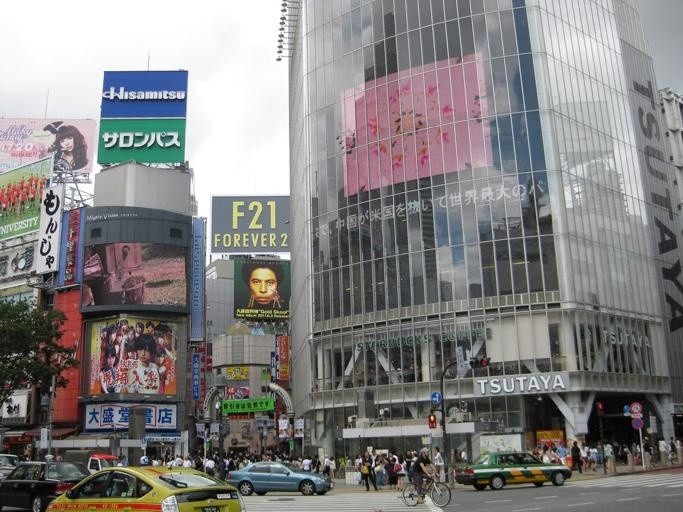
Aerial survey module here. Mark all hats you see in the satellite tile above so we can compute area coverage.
[421,448,430,452]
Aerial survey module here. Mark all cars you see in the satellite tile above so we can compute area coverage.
[226,461,333,495]
[0,461,91,512]
[42,464,244,512]
[454,451,573,490]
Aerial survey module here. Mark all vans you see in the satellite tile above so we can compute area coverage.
[0,453,20,484]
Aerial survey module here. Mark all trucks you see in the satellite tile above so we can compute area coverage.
[63,447,120,477]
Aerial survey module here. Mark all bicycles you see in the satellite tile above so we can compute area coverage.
[402,471,452,509]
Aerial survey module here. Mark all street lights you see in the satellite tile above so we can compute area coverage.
[215,385,228,481]
[41,396,54,461]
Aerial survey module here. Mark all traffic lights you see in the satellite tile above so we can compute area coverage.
[429,413,436,428]
[594,401,604,418]
[469,358,490,368]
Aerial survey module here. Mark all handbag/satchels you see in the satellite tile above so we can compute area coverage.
[311,468,317,473]
[394,464,402,473]
[363,466,368,473]
[437,458,443,465]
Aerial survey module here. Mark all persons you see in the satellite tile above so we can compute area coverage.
[241,260,286,318]
[114,446,470,490]
[52,125,88,170]
[526,435,682,475]
[408,447,438,503]
[116,245,130,270]
[18,448,66,466]
[0,172,47,222]
[92,318,176,396]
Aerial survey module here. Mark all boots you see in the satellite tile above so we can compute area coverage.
[418,495,426,503]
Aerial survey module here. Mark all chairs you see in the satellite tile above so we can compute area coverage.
[85,478,110,495]
[109,477,131,497]
